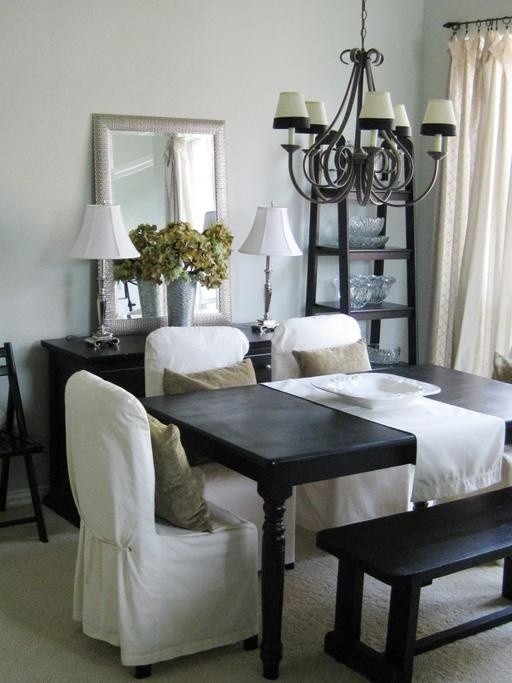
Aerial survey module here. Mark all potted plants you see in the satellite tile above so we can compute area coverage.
[143,216,234,328]
[112,221,167,318]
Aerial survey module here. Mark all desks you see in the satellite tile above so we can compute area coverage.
[40,316,273,529]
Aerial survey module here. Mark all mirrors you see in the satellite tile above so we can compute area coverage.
[90,111,234,338]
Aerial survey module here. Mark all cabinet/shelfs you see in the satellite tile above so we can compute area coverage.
[303,129,420,363]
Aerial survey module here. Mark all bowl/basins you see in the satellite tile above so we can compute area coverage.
[311,372,441,409]
[367,344,401,365]
[347,215,387,235]
[332,274,396,308]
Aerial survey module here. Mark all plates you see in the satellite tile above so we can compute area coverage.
[347,234,389,247]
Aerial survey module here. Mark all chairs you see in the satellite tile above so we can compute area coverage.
[0,339,51,545]
[64,364,262,679]
[144,325,297,572]
[266,311,373,381]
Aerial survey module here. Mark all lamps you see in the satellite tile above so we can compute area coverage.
[238,199,305,335]
[272,0,459,209]
[69,199,143,347]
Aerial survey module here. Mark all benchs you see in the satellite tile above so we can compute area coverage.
[313,485,510,683]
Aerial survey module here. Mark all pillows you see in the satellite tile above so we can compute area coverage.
[147,411,217,533]
[163,356,257,395]
[291,336,372,378]
